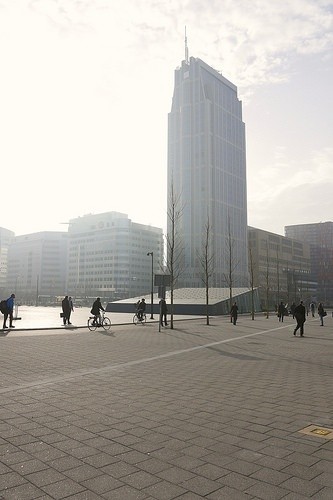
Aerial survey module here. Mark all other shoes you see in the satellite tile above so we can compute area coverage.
[1,319,326,338]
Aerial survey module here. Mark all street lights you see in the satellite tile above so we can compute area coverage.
[147,250,155,320]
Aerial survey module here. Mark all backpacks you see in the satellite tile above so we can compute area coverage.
[0,297,11,314]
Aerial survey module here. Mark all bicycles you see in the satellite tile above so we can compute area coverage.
[133,308,146,325]
[88,309,111,331]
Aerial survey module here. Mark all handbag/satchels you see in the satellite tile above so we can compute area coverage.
[277,313,280,318]
[60,313,66,317]
[323,312,327,316]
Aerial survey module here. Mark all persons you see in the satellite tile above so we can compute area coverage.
[230,302,238,325]
[159,298,169,327]
[62,296,71,325]
[68,297,74,319]
[3,294,15,328]
[277,301,324,337]
[135,299,146,321]
[90,298,105,327]
